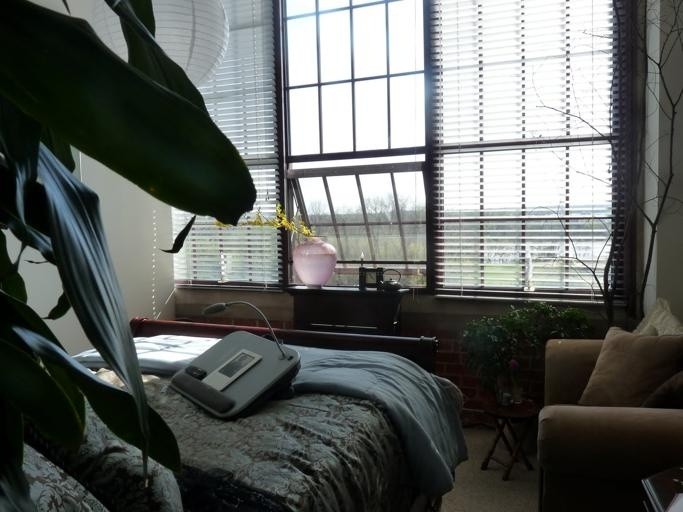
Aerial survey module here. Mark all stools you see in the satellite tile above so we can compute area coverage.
[480,401,541,482]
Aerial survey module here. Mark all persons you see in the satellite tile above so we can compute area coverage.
[220,354,247,377]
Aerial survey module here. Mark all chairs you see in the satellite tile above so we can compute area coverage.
[537,337,682,512]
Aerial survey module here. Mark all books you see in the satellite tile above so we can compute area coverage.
[640,468,683,512]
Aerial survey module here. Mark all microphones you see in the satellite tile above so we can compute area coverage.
[200,300,286,359]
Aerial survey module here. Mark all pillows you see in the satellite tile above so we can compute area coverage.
[577,326,683,408]
[18,408,186,510]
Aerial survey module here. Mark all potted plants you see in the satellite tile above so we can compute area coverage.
[215,204,337,288]
[462,300,595,452]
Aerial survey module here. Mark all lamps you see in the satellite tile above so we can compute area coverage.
[201,300,286,360]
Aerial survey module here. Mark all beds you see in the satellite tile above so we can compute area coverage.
[44,316,439,512]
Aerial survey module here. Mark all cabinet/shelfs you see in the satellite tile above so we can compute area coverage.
[284,283,409,333]
